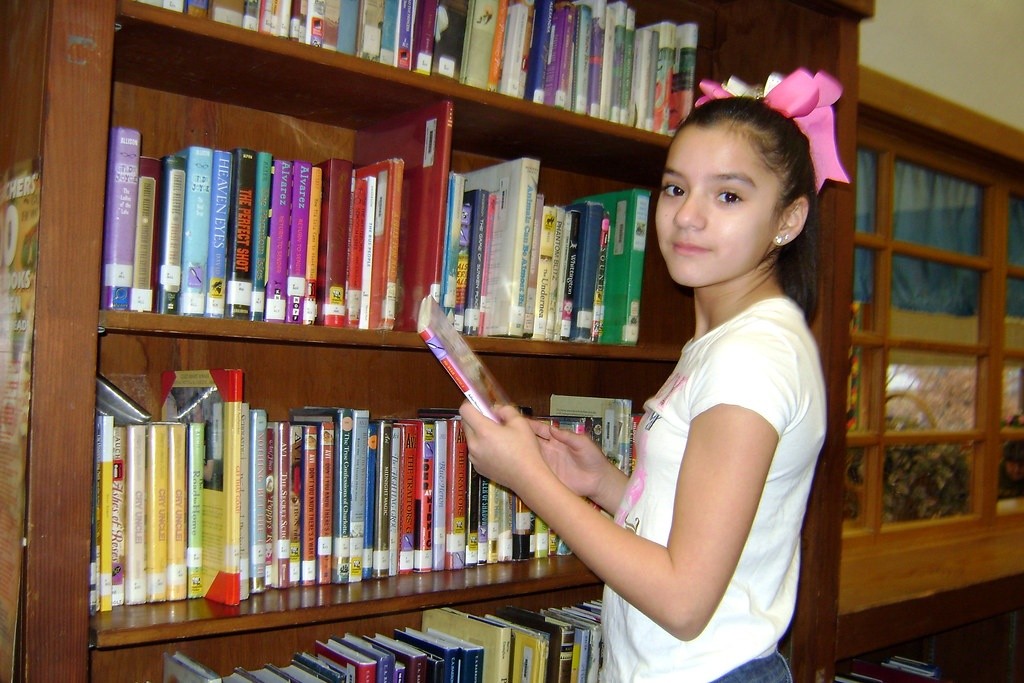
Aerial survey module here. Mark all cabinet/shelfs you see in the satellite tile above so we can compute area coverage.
[0,1,873,683]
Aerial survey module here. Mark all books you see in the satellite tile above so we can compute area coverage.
[88,0,942,683]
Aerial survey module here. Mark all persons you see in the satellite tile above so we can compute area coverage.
[458,67,850,683]
